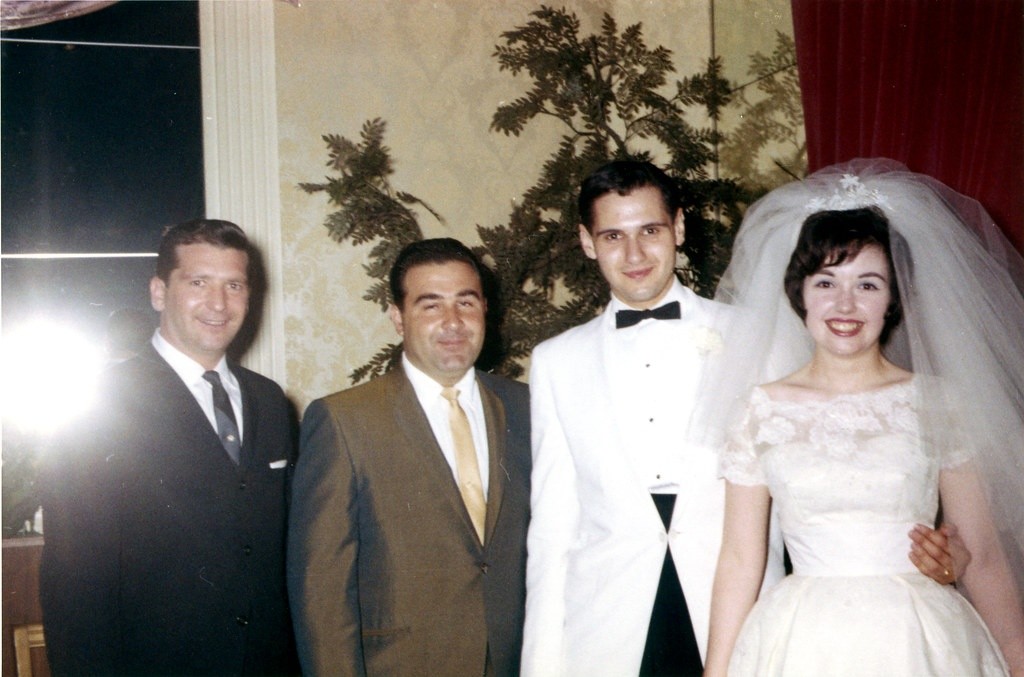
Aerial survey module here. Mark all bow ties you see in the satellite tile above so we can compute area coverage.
[615,301,682,332]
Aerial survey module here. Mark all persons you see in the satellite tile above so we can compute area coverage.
[38,218,298,677]
[287,236,530,677]
[521,156,1024,677]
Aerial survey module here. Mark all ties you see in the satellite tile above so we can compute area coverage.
[202,370,240,473]
[441,385,486,547]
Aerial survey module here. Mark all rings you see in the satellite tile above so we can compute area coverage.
[937,569,950,578]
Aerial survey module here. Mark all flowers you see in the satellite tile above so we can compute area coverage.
[807,174,888,210]
